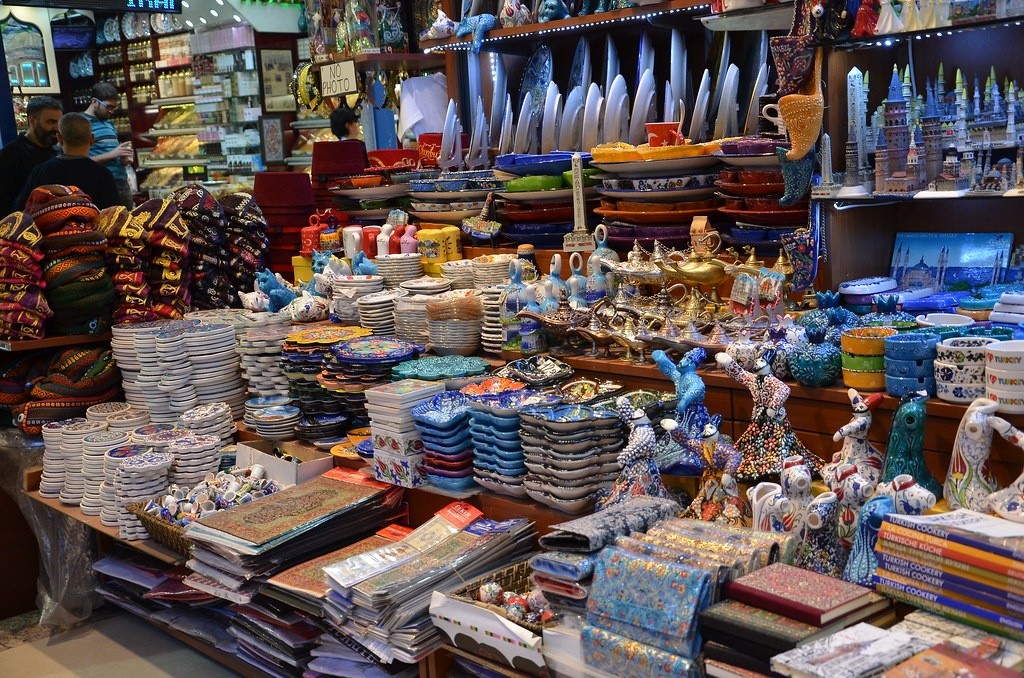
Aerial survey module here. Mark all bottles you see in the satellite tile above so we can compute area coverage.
[516,243,544,279]
[98,46,123,65]
[131,83,157,103]
[247,96,252,108]
[99,67,125,87]
[158,67,196,98]
[129,62,154,82]
[127,40,152,61]
[111,115,130,132]
[116,90,128,110]
[72,87,92,105]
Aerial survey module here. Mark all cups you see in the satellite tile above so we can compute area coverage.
[300,224,382,260]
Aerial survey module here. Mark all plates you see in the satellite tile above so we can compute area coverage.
[69,52,94,79]
[500,230,781,257]
[328,184,504,201]
[495,207,808,225]
[150,13,183,34]
[340,209,482,223]
[440,22,768,169]
[121,12,150,40]
[95,16,120,45]
[331,253,520,357]
[492,156,592,176]
[588,152,778,178]
[242,395,374,469]
[410,355,626,515]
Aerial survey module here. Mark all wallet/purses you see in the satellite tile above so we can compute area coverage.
[528,493,794,678]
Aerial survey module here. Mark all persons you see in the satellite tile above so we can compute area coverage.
[80,84,135,212]
[330,108,360,142]
[0,98,120,221]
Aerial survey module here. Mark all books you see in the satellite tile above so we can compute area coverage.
[699,508,1024,678]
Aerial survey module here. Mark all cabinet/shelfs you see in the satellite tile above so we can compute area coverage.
[0,0,1024,678]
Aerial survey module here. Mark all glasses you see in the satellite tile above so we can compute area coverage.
[94,98,118,111]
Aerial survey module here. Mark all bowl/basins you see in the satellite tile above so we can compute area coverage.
[502,201,573,234]
[337,198,486,212]
[409,170,503,191]
[590,132,793,192]
[334,168,439,189]
[598,199,807,241]
[492,150,602,190]
